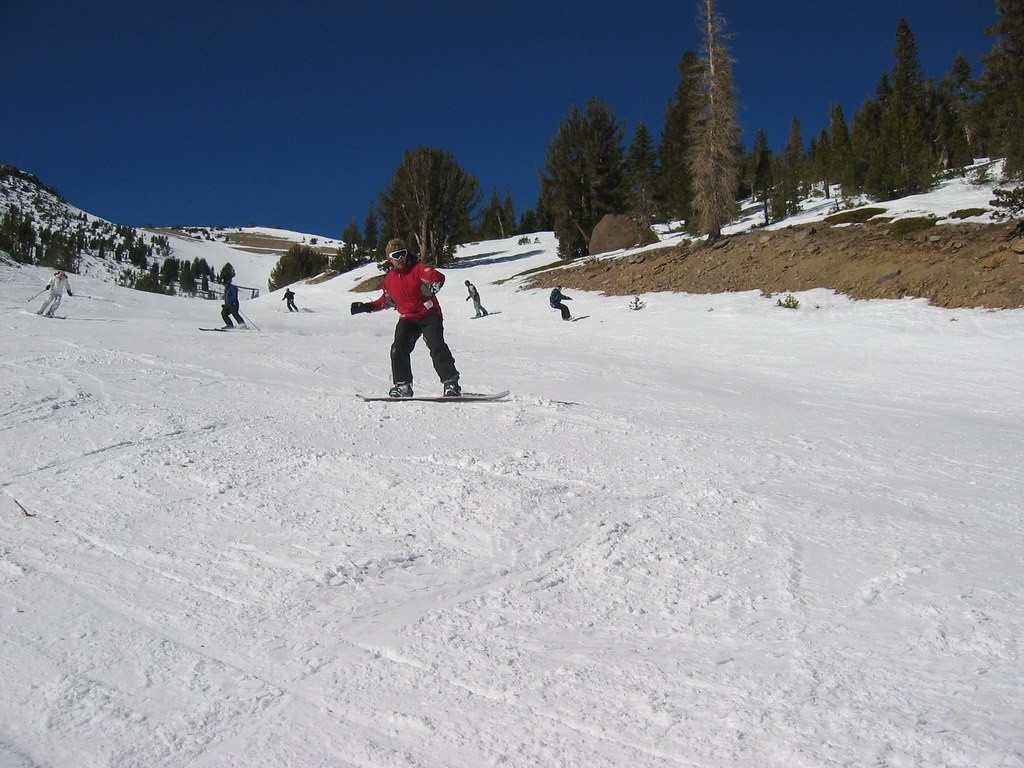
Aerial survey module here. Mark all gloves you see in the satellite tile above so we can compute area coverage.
[46,285,50,290]
[67,290,73,296]
[351,302,373,315]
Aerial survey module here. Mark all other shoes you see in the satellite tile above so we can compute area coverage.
[222,325,234,329]
[476,313,481,317]
[483,312,488,315]
[236,324,246,328]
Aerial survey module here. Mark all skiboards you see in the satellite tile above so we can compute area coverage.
[198,327,265,332]
[283,311,300,313]
[34,311,67,319]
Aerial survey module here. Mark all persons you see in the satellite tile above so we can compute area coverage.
[550,286,572,320]
[221,277,247,329]
[38,270,73,318]
[282,288,299,311]
[351,239,460,396]
[465,280,489,316]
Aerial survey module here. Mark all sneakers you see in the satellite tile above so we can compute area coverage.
[444,380,461,396]
[389,382,413,397]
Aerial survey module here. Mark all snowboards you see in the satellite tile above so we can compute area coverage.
[355,390,511,401]
[470,311,502,319]
[570,316,590,322]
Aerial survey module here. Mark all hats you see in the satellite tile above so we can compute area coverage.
[386,238,409,263]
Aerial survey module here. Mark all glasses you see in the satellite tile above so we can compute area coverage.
[389,249,408,261]
